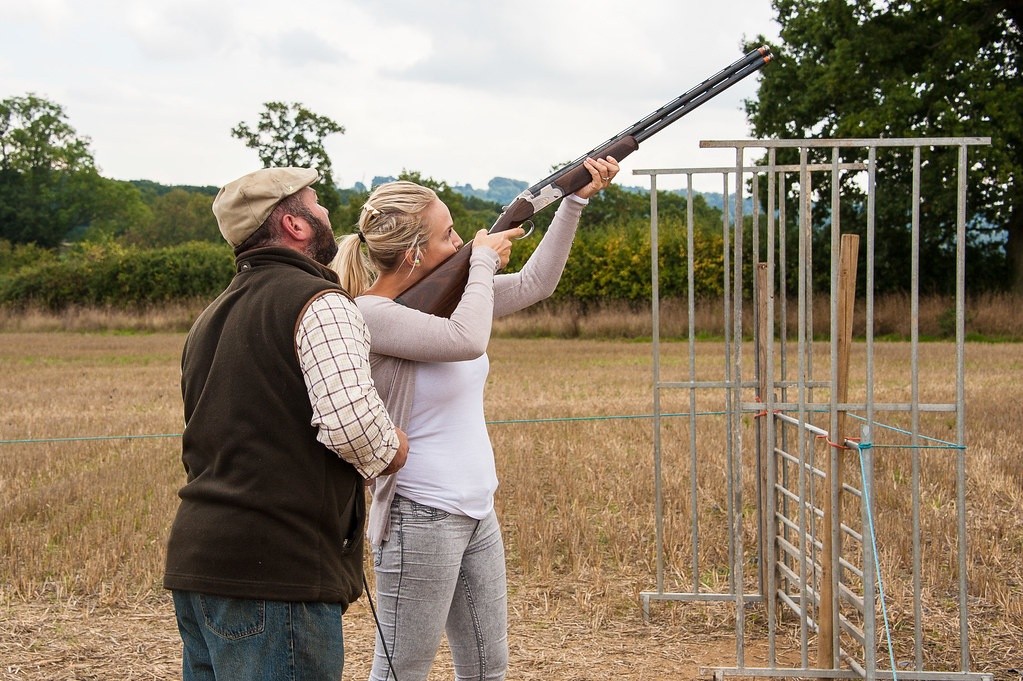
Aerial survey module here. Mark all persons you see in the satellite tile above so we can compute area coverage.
[328,157,619,681]
[164,167,411,681]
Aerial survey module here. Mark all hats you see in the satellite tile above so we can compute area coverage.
[211,166,319,249]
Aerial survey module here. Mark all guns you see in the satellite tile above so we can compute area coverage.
[396,46,775,319]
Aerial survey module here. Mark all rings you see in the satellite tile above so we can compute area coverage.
[602,178,609,180]
